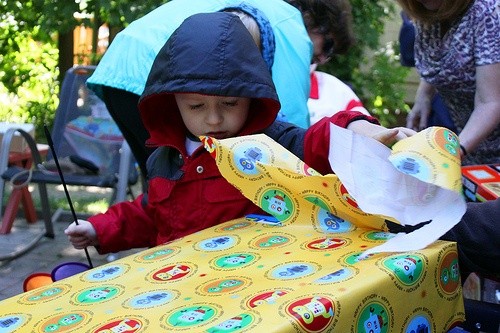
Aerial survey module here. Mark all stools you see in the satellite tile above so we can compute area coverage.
[1,143,50,235]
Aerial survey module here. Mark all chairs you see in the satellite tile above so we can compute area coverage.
[2,66,144,263]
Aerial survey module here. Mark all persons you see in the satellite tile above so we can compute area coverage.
[83,1,355,188]
[217,1,290,123]
[306,64,374,127]
[63,12,421,256]
[385,195,500,273]
[396,0,500,167]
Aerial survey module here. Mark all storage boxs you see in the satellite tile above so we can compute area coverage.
[0,217,466,333]
[460,163,500,204]
[0,121,35,151]
